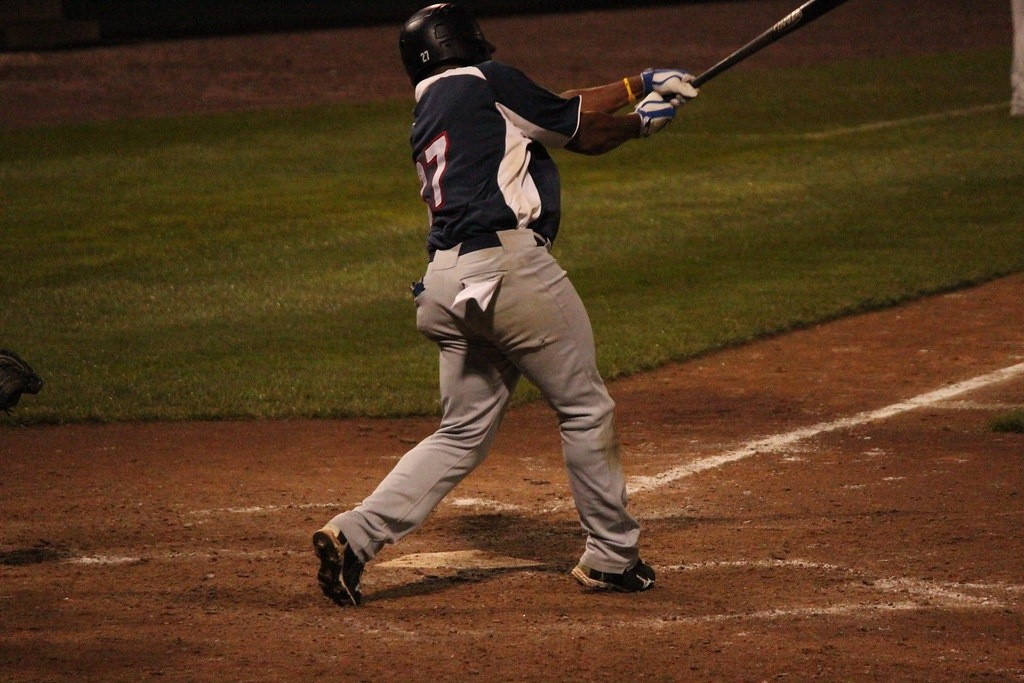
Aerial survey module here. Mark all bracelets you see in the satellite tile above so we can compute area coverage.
[623,77,637,105]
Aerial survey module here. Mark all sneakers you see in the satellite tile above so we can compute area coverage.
[312,522,365,608]
[572,557,657,593]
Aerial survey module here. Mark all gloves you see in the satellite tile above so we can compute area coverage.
[640,68,701,108]
[626,90,676,139]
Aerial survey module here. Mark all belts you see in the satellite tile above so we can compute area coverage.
[427,233,545,264]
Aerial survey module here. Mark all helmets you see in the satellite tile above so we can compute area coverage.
[399,4,489,85]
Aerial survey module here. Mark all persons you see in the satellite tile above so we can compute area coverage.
[313,4,699,608]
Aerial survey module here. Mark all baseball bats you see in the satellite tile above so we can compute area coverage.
[662,0,846,102]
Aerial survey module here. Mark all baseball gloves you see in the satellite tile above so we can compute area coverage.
[0,348,45,411]
